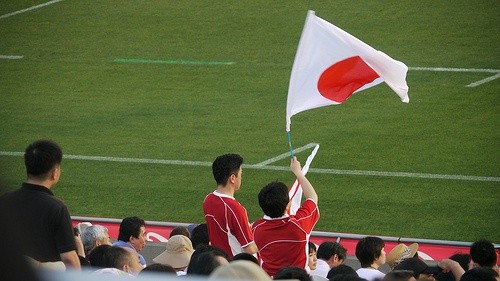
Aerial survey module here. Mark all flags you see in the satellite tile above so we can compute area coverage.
[285,10,410,132]
[287,178,306,215]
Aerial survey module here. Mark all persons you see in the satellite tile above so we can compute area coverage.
[0,140,80,272]
[251,156,320,277]
[0,216,500,281]
[203,152,259,264]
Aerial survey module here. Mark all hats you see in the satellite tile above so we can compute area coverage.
[75,222,92,234]
[394,258,442,277]
[208,260,300,281]
[153,235,196,269]
[386,243,418,271]
